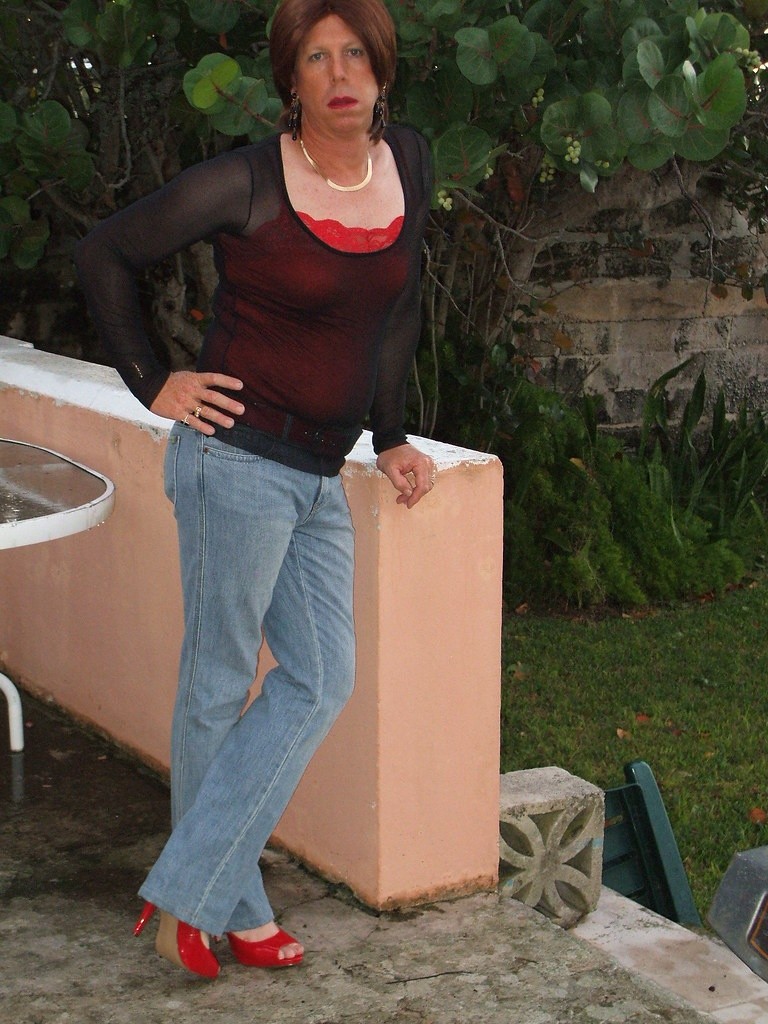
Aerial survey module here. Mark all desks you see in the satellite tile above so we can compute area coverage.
[1,438,118,753]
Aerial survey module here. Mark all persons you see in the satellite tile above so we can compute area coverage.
[73,0,437,978]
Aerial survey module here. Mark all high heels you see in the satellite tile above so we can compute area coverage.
[209,919,305,967]
[134,901,222,980]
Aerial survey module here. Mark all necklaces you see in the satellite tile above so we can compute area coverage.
[300,133,374,191]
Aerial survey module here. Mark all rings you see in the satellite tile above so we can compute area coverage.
[180,411,192,427]
[194,403,203,416]
[429,469,437,486]
